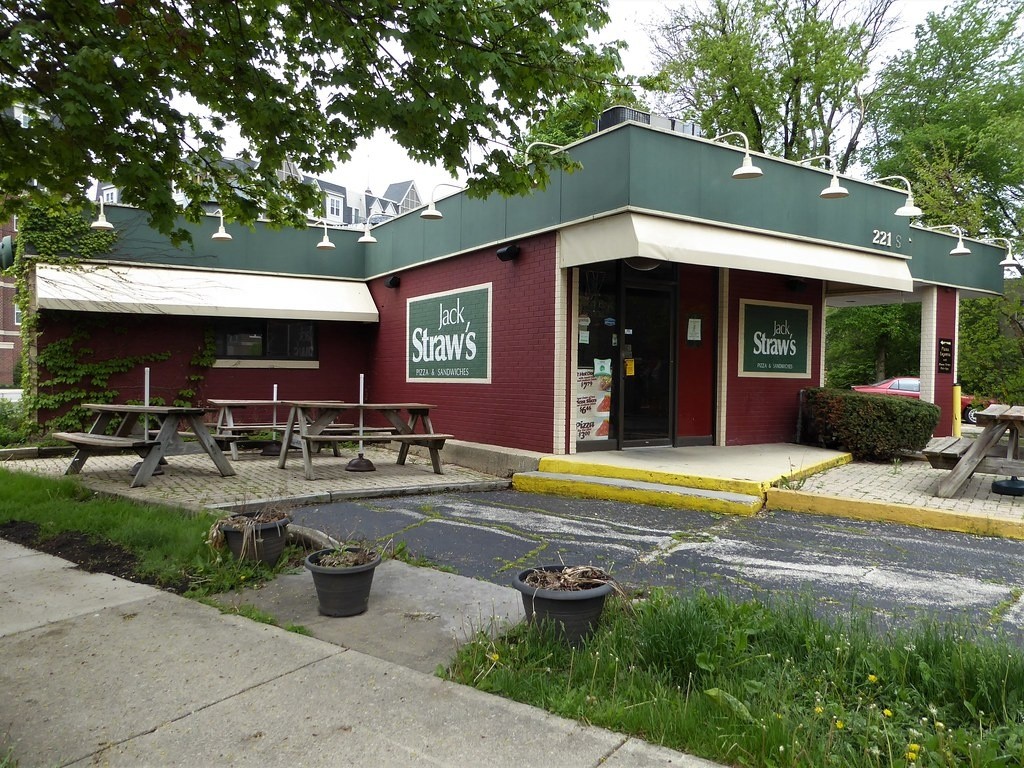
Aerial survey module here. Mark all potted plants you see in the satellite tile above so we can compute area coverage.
[304,531,382,618]
[206,501,296,571]
[514,564,637,648]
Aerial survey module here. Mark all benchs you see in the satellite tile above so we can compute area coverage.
[922,436,975,469]
[274,427,395,435]
[302,434,455,441]
[51,433,163,459]
[218,424,354,430]
[149,430,248,451]
[204,422,319,427]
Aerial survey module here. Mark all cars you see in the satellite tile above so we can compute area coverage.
[852,377,1000,425]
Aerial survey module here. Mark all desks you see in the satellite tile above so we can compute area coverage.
[934,405,1024,499]
[65,399,446,488]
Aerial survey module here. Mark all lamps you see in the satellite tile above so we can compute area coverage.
[982,237,1018,266]
[421,183,463,219]
[872,175,923,217]
[712,132,764,179]
[929,225,972,255]
[357,214,394,244]
[212,209,233,240]
[797,155,850,198]
[315,220,337,248]
[90,196,115,230]
